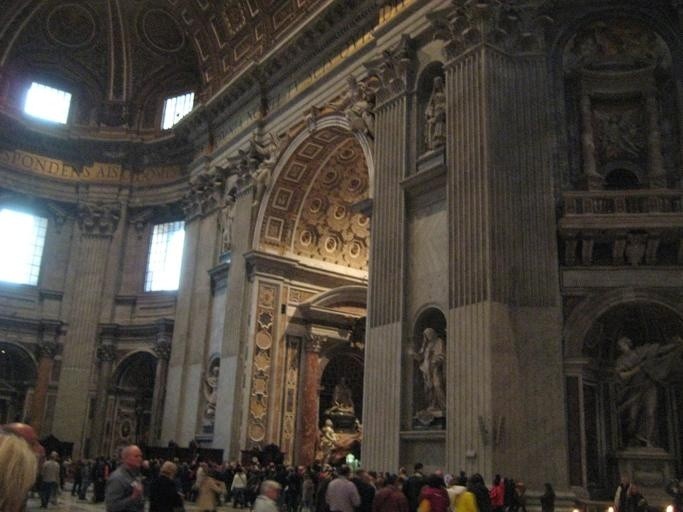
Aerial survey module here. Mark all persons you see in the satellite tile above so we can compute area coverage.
[0,433,37,512]
[221,195,234,251]
[665,479,683,512]
[416,328,446,410]
[615,337,683,448]
[614,476,633,512]
[331,377,354,414]
[203,366,219,424]
[249,150,275,207]
[330,74,375,139]
[619,484,648,512]
[594,111,635,160]
[0,421,46,462]
[424,76,446,149]
[320,419,336,464]
